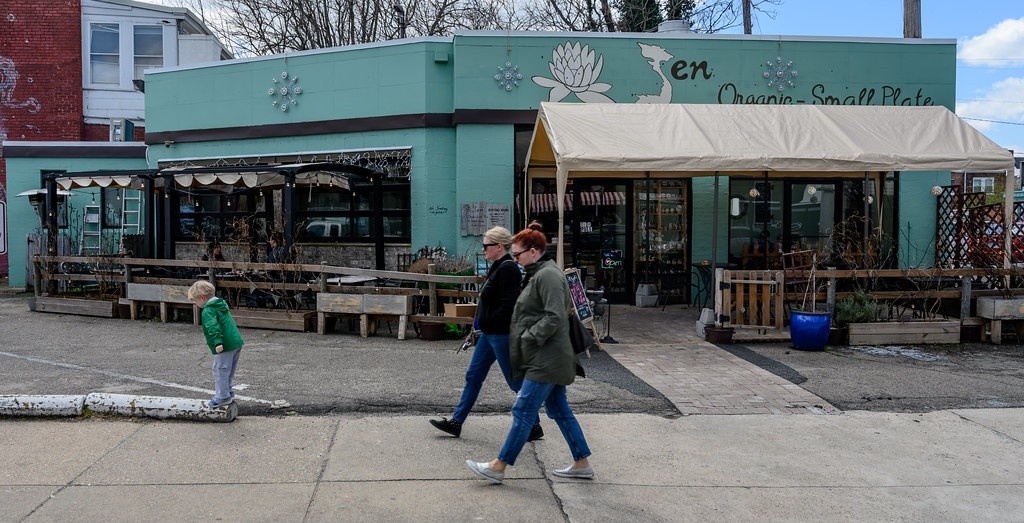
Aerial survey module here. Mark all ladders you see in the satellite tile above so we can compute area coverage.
[79,205,102,255]
[120,187,142,242]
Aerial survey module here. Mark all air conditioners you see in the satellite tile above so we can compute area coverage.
[109,117,134,141]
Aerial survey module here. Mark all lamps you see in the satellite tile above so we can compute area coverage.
[132,80,144,94]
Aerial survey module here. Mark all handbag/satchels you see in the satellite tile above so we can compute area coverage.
[471,330,482,346]
[567,312,596,356]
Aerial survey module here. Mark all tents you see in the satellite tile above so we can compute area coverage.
[519,103,1018,296]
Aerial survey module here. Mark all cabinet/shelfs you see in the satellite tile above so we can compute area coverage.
[633,179,687,302]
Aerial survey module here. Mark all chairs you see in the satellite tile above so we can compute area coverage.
[653,256,701,312]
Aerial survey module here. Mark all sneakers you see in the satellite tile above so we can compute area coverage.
[208,391,235,407]
[465,460,505,484]
[429,418,462,438]
[527,424,544,442]
[553,464,594,477]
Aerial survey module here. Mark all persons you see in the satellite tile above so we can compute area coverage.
[428,225,546,443]
[201,234,294,308]
[466,220,594,485]
[406,257,435,274]
[188,280,245,407]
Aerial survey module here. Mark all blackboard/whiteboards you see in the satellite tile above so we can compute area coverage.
[564,269,594,324]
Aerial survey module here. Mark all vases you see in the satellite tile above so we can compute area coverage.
[789,308,831,351]
[976,295,1024,320]
[705,325,733,343]
[229,306,317,333]
[419,322,445,340]
[117,305,141,318]
[28,296,117,318]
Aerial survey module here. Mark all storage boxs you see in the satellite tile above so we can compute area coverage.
[444,303,478,317]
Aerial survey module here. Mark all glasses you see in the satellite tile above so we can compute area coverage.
[511,248,531,257]
[482,242,499,252]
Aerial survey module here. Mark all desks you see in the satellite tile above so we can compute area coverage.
[196,270,269,282]
[689,262,739,321]
[310,275,385,331]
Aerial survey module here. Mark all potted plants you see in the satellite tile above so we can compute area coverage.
[835,289,961,346]
